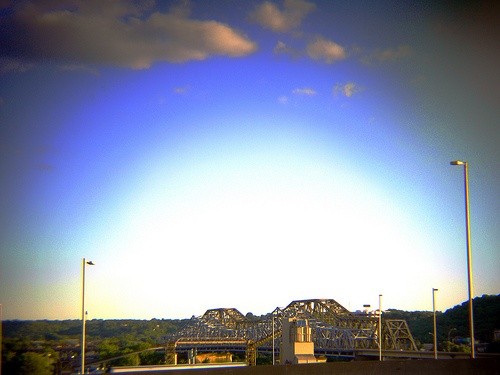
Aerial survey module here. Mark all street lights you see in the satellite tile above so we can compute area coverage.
[450,159,478,358]
[271,313,278,365]
[80,257,94,375]
[432,286,443,359]
[378,291,386,361]
